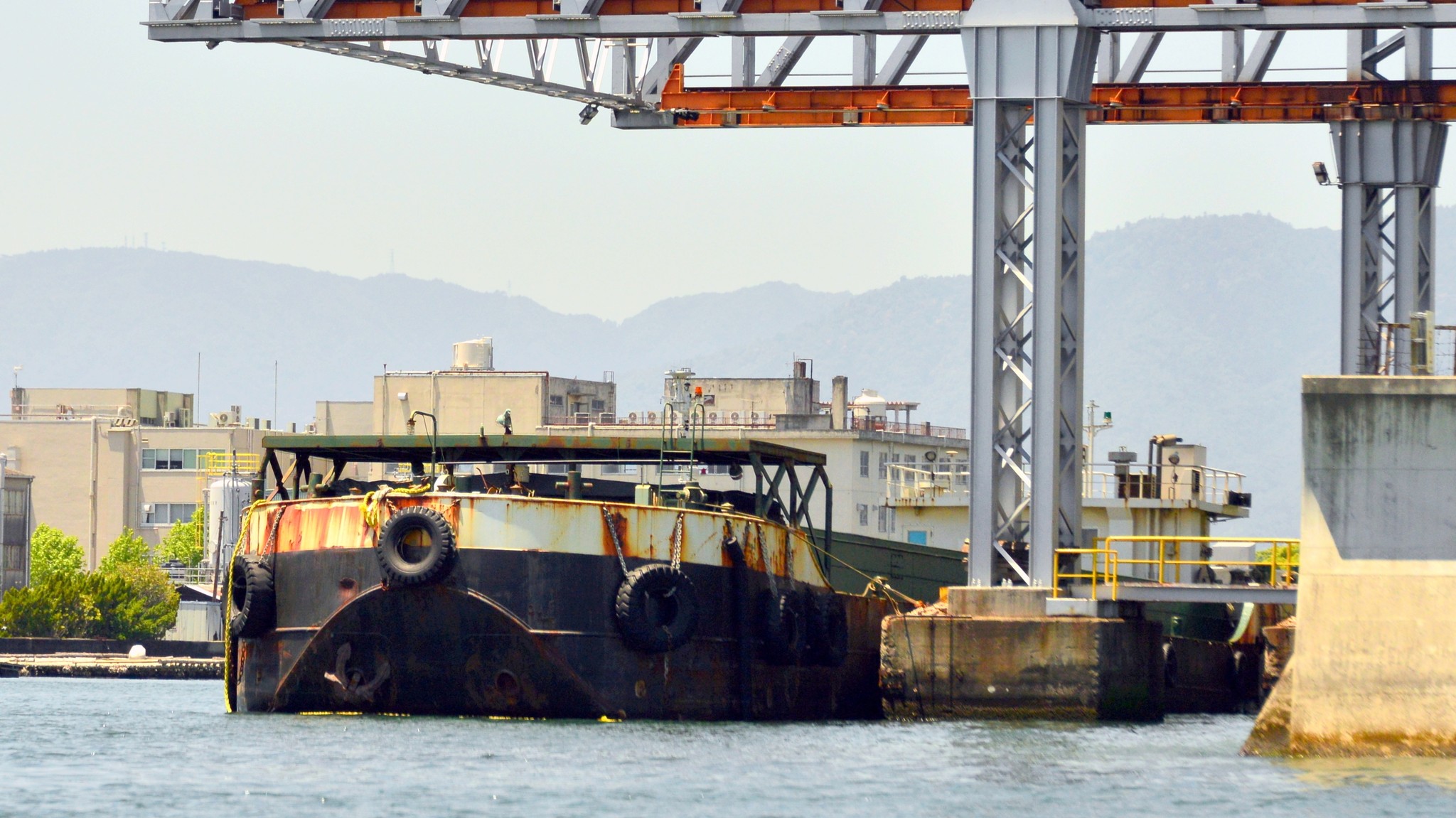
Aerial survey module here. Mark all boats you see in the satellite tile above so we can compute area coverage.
[218,402,1267,726]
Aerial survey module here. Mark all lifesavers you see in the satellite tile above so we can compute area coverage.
[766,587,806,663]
[614,564,698,652]
[219,554,277,639]
[377,505,457,587]
[1232,650,1248,685]
[1148,641,1179,705]
[808,591,849,665]
